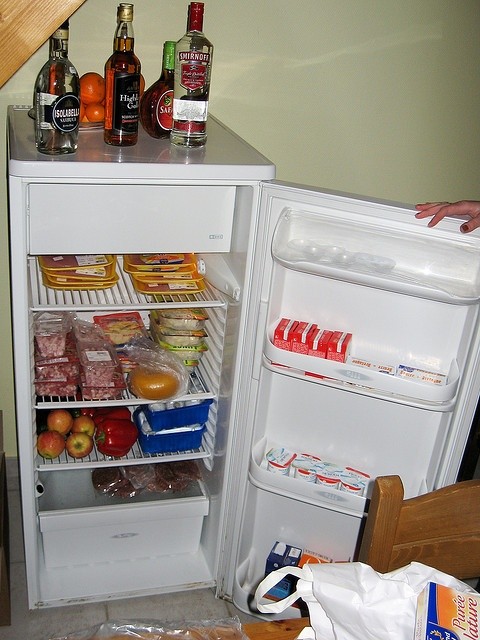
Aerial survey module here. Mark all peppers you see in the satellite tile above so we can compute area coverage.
[94,418,139,457]
[93,405,132,428]
[80,408,99,417]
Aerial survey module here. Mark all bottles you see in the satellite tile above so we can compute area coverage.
[168,1,215,150]
[103,2,142,148]
[141,40,175,139]
[32,18,81,156]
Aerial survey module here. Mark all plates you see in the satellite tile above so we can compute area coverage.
[27,106,109,132]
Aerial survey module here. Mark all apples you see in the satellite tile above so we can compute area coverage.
[65,432,94,459]
[37,430,65,459]
[70,415,96,439]
[46,409,73,436]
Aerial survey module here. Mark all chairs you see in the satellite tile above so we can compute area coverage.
[355,476,480,588]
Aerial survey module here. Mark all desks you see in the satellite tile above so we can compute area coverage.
[110,617,312,639]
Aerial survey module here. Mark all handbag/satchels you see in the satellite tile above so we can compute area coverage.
[250,556,477,640]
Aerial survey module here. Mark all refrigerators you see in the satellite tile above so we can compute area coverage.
[6,102,480,621]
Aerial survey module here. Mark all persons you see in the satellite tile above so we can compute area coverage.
[414,199,479,234]
